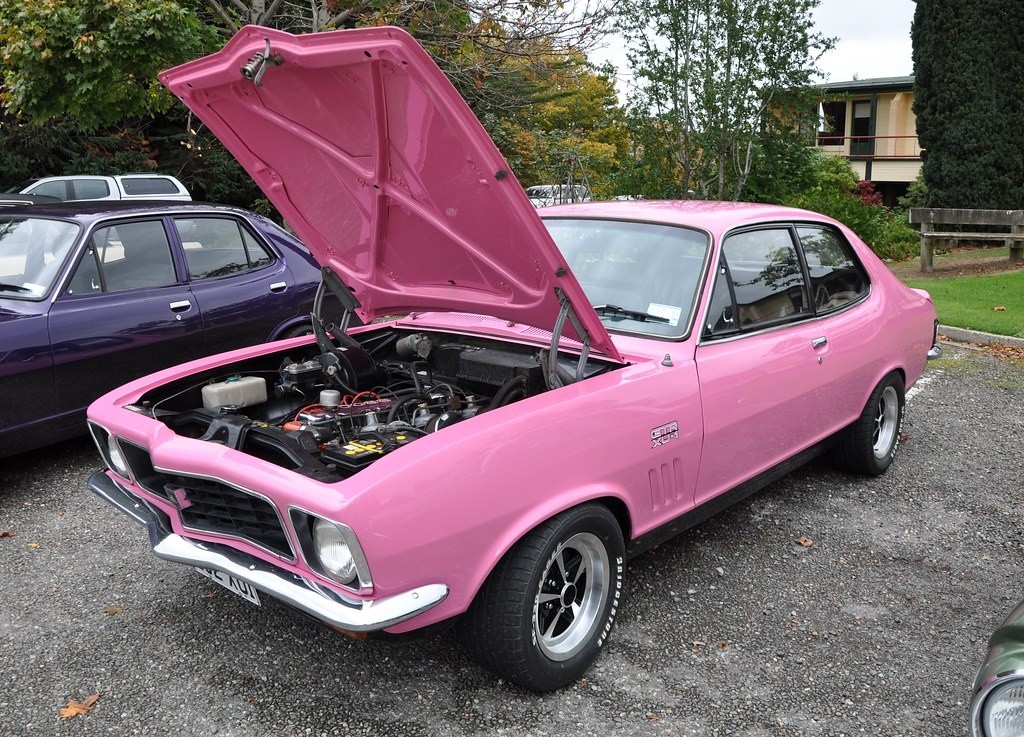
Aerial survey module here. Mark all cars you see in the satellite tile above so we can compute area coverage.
[522,181,592,209]
[83,22,942,699]
[1,193,204,278]
[0,204,367,462]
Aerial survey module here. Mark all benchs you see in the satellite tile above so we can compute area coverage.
[910,205,1024,242]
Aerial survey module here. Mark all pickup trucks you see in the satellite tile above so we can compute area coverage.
[1,167,192,202]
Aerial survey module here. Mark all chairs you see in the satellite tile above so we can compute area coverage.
[39,234,88,287]
[718,250,795,326]
[110,239,172,289]
[636,242,695,317]
